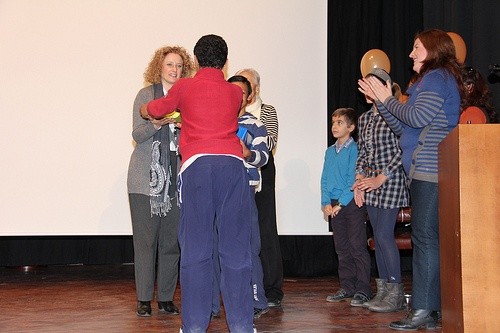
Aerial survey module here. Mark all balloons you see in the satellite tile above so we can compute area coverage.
[446,31,466,64]
[361,49,391,76]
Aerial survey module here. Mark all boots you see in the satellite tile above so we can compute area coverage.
[369,283,405,312]
[362,279,389,308]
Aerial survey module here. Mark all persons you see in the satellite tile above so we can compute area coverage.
[127,46,283,319]
[320,30,496,331]
[139,35,258,333]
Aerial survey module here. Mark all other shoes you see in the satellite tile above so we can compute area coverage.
[326,289,352,302]
[351,295,367,307]
[254,306,270,318]
[210,314,221,321]
[267,299,280,307]
[180,325,184,333]
[253,323,257,333]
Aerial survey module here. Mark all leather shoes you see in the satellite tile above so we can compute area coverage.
[136,300,152,316]
[157,300,179,314]
[388,308,442,330]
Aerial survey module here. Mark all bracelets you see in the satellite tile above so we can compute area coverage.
[355,179,359,182]
[335,202,344,207]
